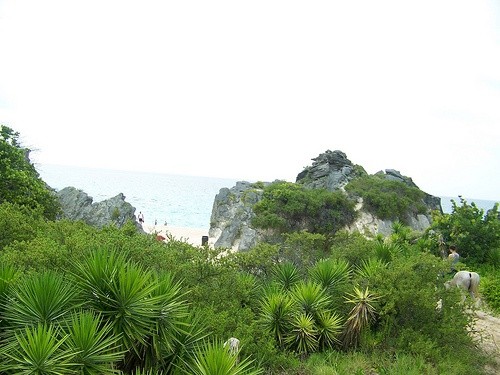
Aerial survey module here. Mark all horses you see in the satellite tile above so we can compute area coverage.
[441,271,482,309]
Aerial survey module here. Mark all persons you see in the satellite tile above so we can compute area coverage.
[138,211,144,224]
[164,221,167,226]
[439,246,460,276]
